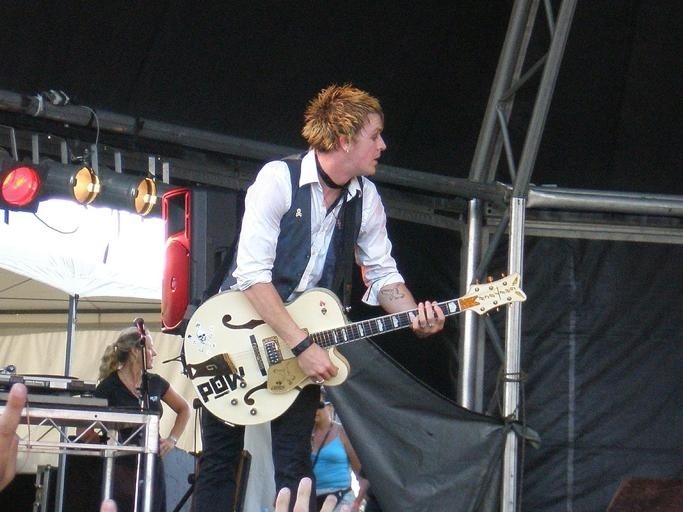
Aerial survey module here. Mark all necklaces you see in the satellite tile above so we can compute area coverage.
[315,155,351,189]
[320,187,352,229]
[118,370,141,394]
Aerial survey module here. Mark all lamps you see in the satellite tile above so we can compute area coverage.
[0,124,157,224]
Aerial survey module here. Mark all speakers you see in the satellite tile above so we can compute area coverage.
[158,184,240,334]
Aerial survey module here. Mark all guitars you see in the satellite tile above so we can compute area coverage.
[183,273,528,427]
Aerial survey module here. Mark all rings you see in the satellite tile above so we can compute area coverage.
[427,322,435,327]
[419,325,428,329]
[315,378,324,384]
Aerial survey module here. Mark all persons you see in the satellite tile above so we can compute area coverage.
[309,390,359,511]
[91,325,193,512]
[183,83,445,511]
[269,474,340,511]
[0,379,28,493]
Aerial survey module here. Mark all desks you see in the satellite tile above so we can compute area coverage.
[0,398,161,511]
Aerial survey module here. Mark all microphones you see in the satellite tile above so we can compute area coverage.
[133,317,147,343]
[318,402,333,407]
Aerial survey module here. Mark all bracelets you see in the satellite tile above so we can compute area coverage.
[167,435,177,445]
[289,333,312,356]
